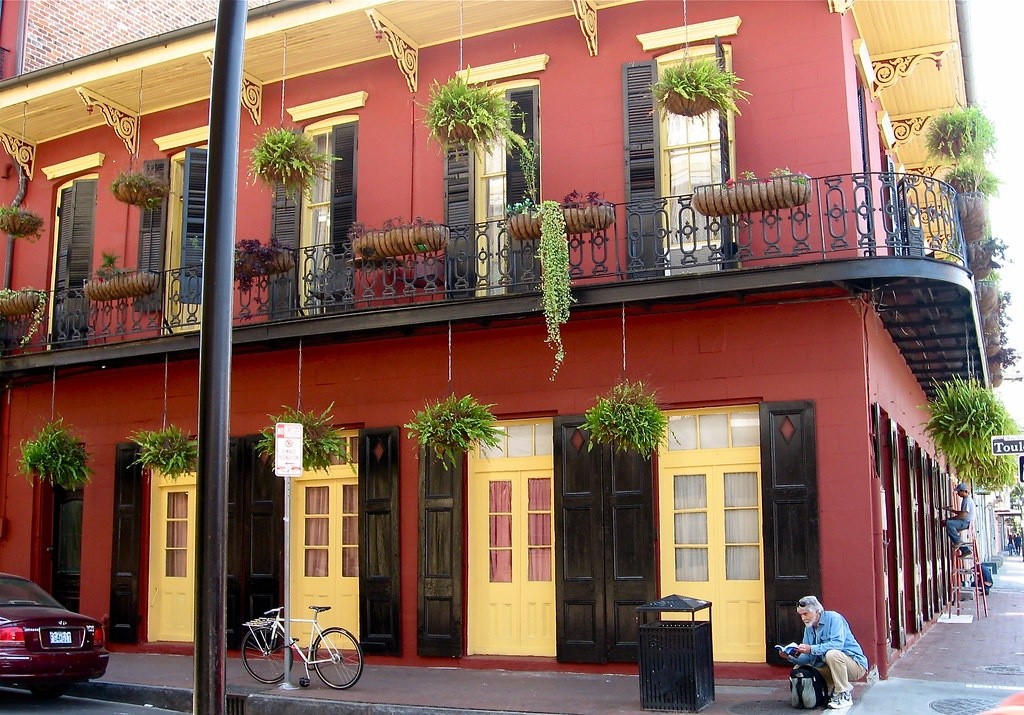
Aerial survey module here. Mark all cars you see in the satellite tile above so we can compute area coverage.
[0,572,110,701]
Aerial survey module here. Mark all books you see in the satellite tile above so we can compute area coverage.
[775,642,801,658]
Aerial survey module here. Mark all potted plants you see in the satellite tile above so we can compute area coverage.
[0,285,49,347]
[233,235,296,293]
[923,104,1024,389]
[349,214,453,259]
[240,123,343,205]
[80,250,160,302]
[109,157,177,212]
[502,188,615,380]
[408,64,543,205]
[645,53,753,125]
[0,200,46,245]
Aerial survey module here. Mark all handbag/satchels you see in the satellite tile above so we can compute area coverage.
[790,666,828,711]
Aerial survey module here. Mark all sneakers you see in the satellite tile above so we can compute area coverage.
[828,691,853,708]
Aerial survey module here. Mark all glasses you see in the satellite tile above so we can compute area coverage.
[796,600,811,608]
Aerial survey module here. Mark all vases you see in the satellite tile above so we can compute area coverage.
[691,180,809,217]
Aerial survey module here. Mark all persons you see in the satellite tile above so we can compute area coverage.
[779,596,868,709]
[1008,534,1021,556]
[946,484,975,558]
[971,565,993,595]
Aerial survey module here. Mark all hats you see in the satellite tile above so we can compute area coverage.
[954,483,968,491]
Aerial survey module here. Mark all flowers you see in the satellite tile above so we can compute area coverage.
[724,164,810,188]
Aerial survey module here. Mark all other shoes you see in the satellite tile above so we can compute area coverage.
[957,551,971,559]
[954,542,963,552]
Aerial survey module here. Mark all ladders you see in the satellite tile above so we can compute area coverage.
[949,522,988,620]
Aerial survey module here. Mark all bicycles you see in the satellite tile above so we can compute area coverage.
[240,606,364,690]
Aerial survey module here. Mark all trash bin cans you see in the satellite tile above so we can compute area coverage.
[634,594,715,711]
